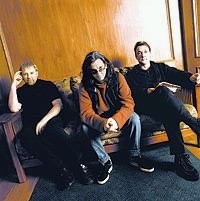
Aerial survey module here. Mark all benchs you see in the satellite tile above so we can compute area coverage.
[0,64,200,183]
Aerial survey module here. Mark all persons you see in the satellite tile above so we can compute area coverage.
[7,60,95,191]
[125,41,200,182]
[79,49,156,184]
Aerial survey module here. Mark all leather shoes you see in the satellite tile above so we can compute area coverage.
[77,162,94,185]
[58,167,75,192]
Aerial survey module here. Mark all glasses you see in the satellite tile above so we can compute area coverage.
[92,65,105,74]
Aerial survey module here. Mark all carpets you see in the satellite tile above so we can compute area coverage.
[28,140,200,201]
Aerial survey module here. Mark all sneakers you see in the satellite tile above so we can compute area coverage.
[128,156,154,173]
[174,154,199,181]
[97,159,113,184]
